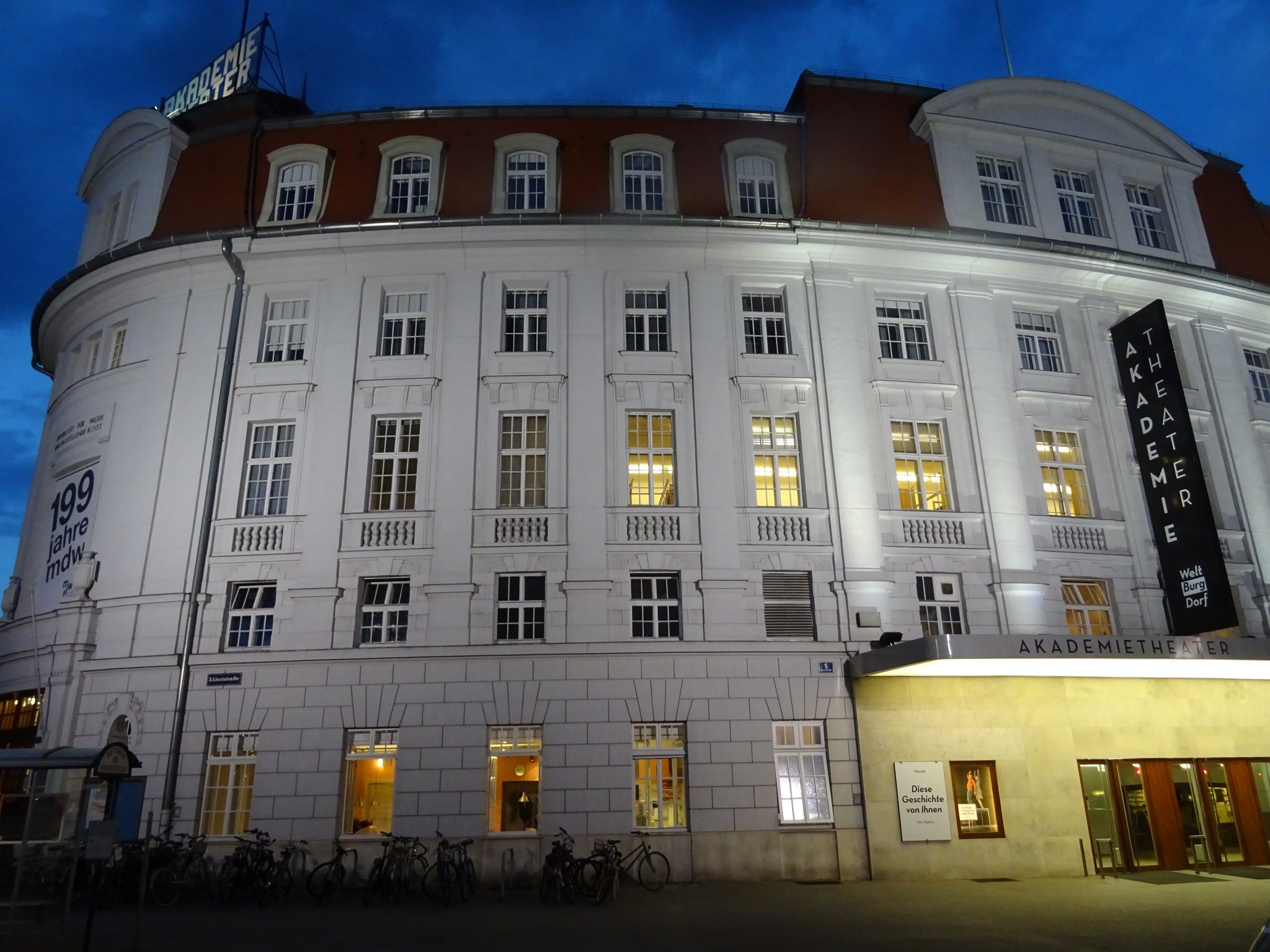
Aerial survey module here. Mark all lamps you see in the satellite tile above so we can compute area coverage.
[518,775,530,802]
[369,768,378,806]
[354,762,364,806]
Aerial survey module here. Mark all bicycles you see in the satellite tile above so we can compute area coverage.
[22,823,308,912]
[306,838,348,901]
[540,826,670,907]
[364,831,478,904]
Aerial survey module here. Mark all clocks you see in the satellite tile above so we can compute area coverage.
[514,764,525,776]
